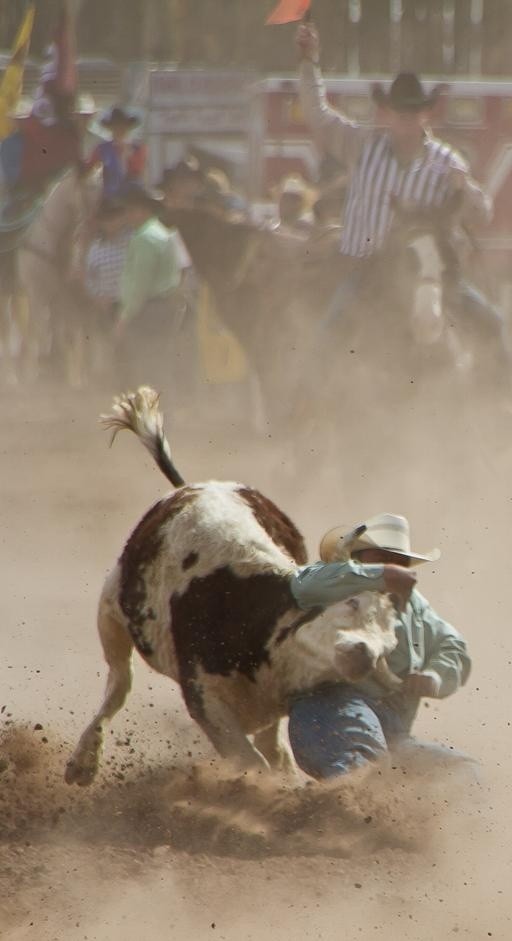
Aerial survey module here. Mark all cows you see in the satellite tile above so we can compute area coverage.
[61,382,407,789]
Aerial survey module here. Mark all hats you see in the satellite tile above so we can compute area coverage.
[274,175,316,207]
[104,185,163,216]
[370,73,451,114]
[99,111,141,132]
[319,514,442,569]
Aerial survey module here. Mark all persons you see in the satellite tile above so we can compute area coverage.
[1,21,509,402]
[288,511,482,808]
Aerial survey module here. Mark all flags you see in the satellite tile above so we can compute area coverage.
[1,0,41,146]
[261,0,314,26]
